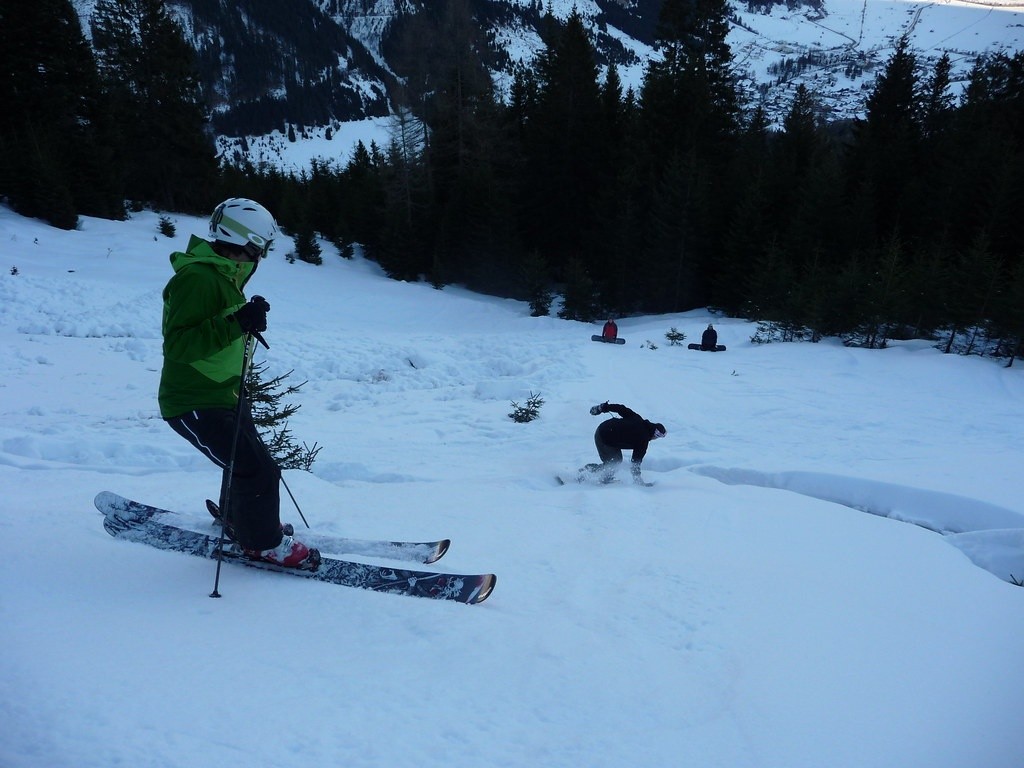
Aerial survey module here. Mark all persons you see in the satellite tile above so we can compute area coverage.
[700,323,718,352]
[602,317,618,343]
[157,198,309,567]
[574,400,666,487]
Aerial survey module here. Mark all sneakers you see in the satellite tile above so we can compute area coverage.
[240,534,309,568]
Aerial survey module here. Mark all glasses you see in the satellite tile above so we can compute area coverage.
[261,239,276,259]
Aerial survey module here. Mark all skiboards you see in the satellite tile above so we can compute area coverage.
[93,490,497,604]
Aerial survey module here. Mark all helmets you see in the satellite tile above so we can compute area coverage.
[208,197,280,257]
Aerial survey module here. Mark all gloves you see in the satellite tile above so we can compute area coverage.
[589,403,605,416]
[234,299,271,334]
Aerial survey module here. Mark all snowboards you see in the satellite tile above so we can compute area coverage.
[591,334,626,346]
[556,473,657,487]
[688,342,727,352]
